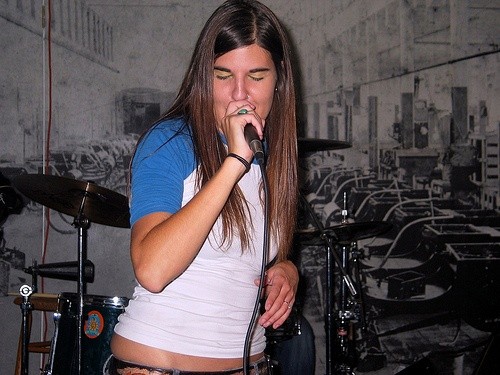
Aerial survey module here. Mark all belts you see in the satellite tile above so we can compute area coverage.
[108,356,272,375]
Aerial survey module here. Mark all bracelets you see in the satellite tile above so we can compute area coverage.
[226,153,251,173]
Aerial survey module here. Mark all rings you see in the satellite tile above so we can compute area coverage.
[237,109,248,115]
[284,301,292,309]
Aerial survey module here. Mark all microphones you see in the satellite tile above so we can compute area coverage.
[244,123,264,163]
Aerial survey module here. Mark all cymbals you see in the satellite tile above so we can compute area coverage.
[297,137,352,152]
[303,221,394,246]
[12,174,131,229]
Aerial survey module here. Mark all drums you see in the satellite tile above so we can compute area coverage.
[261,295,301,337]
[42,291,133,375]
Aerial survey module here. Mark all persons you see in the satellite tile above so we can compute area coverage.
[102,0,301,375]
